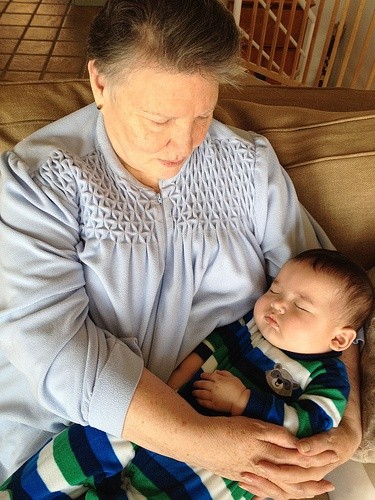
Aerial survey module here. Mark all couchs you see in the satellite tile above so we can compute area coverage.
[0,76,375,484]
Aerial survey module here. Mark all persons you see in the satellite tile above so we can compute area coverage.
[0,1,367,500]
[0,247,375,500]
